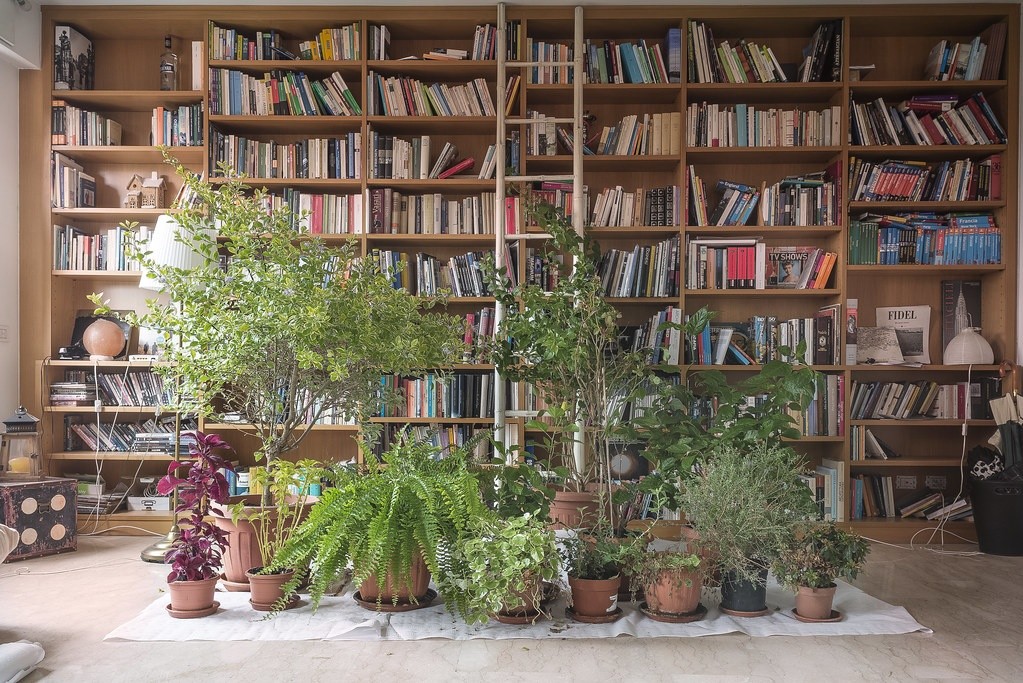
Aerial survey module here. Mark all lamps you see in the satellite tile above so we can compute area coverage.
[139,214,220,565]
[0,405,45,482]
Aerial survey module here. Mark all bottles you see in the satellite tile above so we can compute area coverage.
[160,35,178,90]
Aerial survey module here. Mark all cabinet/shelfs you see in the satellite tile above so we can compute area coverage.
[19,0,1023,546]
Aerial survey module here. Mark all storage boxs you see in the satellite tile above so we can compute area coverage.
[0,478,78,563]
[64,474,106,495]
[127,497,173,511]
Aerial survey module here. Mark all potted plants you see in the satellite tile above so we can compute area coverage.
[86,144,874,624]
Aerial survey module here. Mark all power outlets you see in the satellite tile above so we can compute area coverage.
[926,475,947,490]
[896,475,916,489]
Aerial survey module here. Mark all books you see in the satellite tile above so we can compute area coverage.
[222,250,362,304]
[441,302,521,365]
[53,224,157,270]
[367,241,519,296]
[608,457,845,523]
[529,18,841,84]
[52,101,122,146]
[192,40,206,92]
[63,414,199,453]
[845,22,1010,522]
[254,371,365,428]
[367,373,519,418]
[209,20,521,236]
[49,369,198,408]
[524,104,844,439]
[171,171,204,209]
[380,422,493,484]
[54,27,93,89]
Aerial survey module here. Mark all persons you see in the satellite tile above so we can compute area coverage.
[149,101,204,147]
[51,151,97,208]
